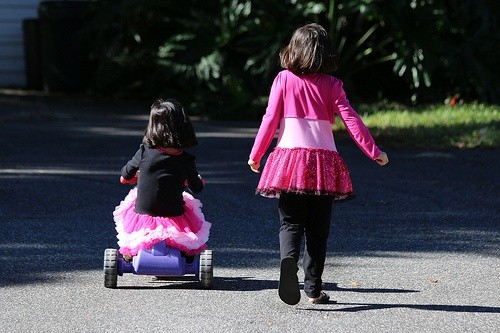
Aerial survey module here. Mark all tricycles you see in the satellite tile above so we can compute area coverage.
[103,174,213,289]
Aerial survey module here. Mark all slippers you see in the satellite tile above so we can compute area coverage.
[308,290,330,304]
[277,256,302,306]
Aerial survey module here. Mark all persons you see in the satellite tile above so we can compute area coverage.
[111,99,213,257]
[246,22,389,305]
